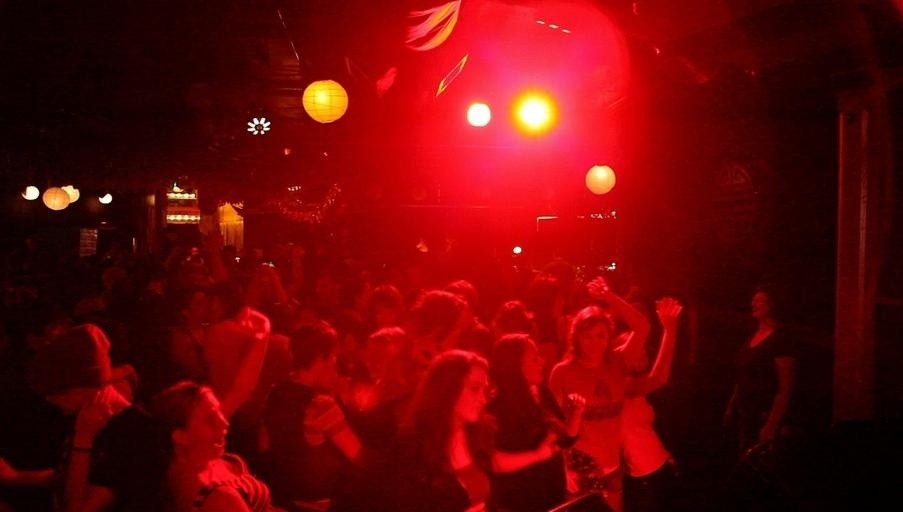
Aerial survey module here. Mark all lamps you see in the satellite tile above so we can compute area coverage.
[18,183,114,213]
[301,78,349,125]
